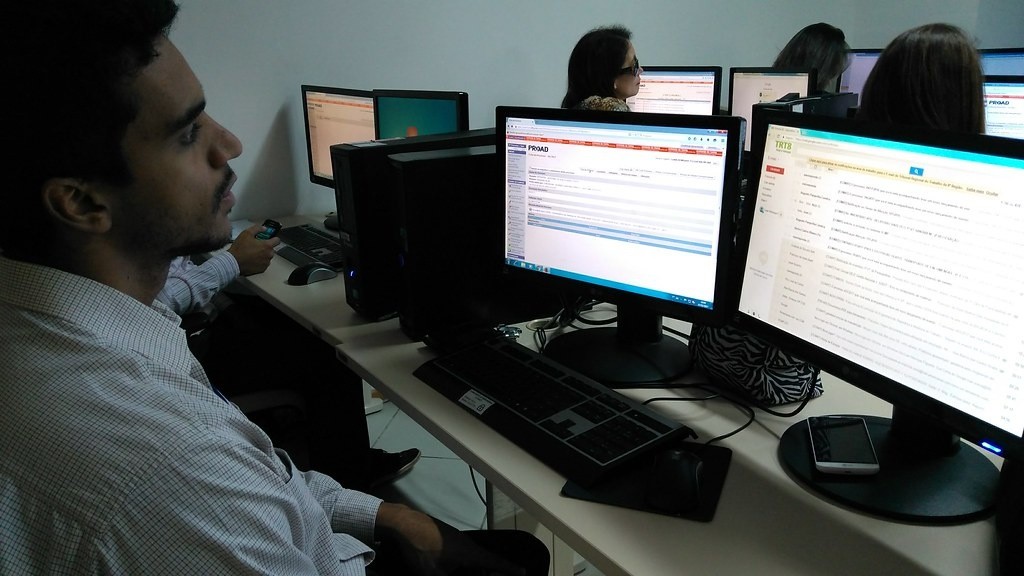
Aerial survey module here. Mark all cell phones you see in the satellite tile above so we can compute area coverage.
[807,416,880,472]
[254,219,283,241]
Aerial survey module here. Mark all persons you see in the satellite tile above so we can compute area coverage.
[155,224,421,489]
[773,22,852,97]
[860,23,985,151]
[561,24,643,112]
[0,0,551,576]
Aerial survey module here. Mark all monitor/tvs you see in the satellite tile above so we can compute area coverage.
[301,84,375,189]
[373,89,469,140]
[624,66,722,115]
[727,66,818,157]
[732,107,1024,528]
[974,48,1024,76]
[835,49,883,107]
[496,106,747,385]
[978,75,1024,140]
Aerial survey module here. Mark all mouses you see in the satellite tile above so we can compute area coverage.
[640,445,700,499]
[288,263,338,286]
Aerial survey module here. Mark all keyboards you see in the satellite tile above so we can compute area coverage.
[411,336,691,484]
[272,223,345,273]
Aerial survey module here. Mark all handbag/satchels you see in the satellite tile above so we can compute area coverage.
[689,322,823,406]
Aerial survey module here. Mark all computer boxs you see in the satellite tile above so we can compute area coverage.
[386,145,593,343]
[329,127,496,322]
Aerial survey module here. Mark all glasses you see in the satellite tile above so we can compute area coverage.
[619,60,640,77]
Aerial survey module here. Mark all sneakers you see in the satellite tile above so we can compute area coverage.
[357,448,421,489]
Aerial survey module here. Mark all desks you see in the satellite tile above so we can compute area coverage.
[202,210,1004,576]
